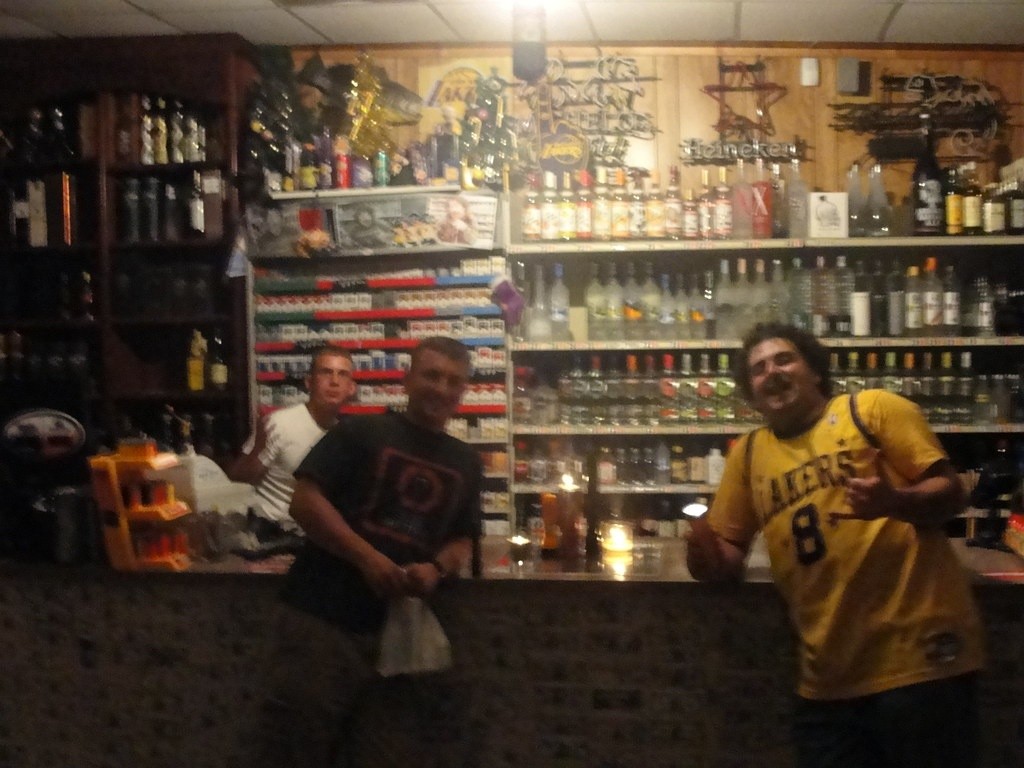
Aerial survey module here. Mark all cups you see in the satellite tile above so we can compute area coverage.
[601,521,635,550]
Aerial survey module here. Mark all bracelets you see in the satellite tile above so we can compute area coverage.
[430,559,447,578]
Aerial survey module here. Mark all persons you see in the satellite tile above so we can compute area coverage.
[224,338,483,768]
[686,321,989,768]
[227,344,357,536]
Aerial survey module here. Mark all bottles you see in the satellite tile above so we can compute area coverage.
[119,169,205,242]
[248,90,1024,240]
[514,353,765,422]
[113,93,209,164]
[993,439,1013,508]
[514,435,734,556]
[831,351,1024,424]
[511,256,1024,340]
[187,329,228,392]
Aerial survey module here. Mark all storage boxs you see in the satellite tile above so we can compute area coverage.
[254,255,509,535]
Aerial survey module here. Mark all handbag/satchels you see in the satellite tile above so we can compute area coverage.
[373,587,452,678]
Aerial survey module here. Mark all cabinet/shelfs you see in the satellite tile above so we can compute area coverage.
[509,236,1024,494]
[247,183,519,538]
[0,32,284,454]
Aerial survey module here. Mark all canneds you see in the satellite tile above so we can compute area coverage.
[372,149,390,186]
[333,153,353,188]
